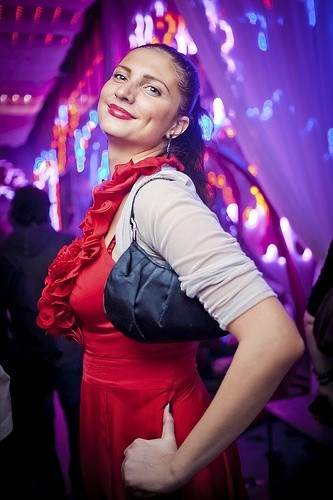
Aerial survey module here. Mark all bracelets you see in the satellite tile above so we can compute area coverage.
[314,366,332,388]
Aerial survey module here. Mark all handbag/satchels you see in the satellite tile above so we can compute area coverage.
[103,177,230,343]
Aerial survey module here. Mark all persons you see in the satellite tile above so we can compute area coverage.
[302,232,333,499]
[35,44,305,500]
[0,185,83,499]
